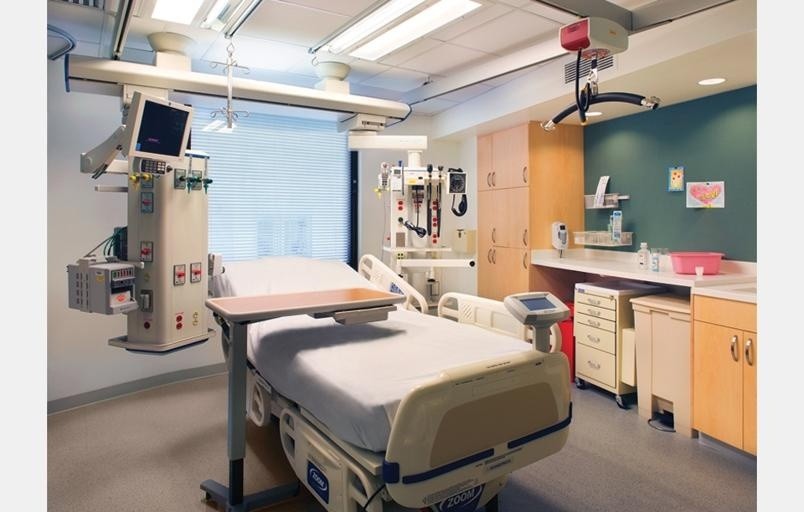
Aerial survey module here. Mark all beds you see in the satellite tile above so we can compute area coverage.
[207,256,573,511]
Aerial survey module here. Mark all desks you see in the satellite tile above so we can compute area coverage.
[199,283,407,512]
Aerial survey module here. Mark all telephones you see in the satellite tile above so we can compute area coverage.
[552,223,569,249]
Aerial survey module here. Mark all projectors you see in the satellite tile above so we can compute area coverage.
[336,114,386,133]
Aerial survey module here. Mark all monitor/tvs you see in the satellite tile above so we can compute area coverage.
[124,90,195,164]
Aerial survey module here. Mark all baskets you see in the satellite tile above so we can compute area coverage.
[670,252,725,274]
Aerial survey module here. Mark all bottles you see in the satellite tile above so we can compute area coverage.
[637,242,660,272]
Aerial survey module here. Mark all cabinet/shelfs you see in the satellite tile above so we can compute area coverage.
[691,295,756,463]
[571,280,637,408]
[475,121,586,307]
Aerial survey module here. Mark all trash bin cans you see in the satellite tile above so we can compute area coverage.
[557,302,575,382]
